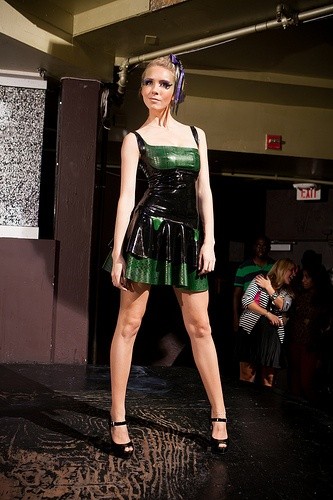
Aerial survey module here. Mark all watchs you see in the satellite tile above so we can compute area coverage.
[270,293,278,300]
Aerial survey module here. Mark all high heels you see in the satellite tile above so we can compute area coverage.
[109,419,133,459]
[209,415,229,455]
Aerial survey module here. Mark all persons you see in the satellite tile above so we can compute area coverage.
[286,259,331,407]
[232,257,299,388]
[100,54,229,456]
[231,235,274,332]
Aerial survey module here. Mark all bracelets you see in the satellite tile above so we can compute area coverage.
[263,310,271,318]
